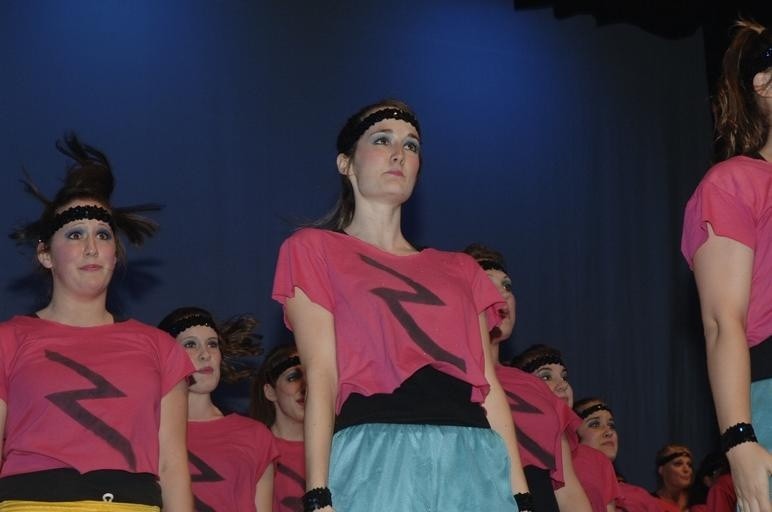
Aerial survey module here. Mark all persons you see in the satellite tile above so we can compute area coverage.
[251,346,305,511]
[458,241,591,511]
[156,307,282,510]
[682,12,770,510]
[271,101,538,511]
[2,131,196,511]
[499,344,738,511]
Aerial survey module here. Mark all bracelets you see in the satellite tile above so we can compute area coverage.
[722,422,758,452]
[303,487,333,511]
[514,492,538,510]
